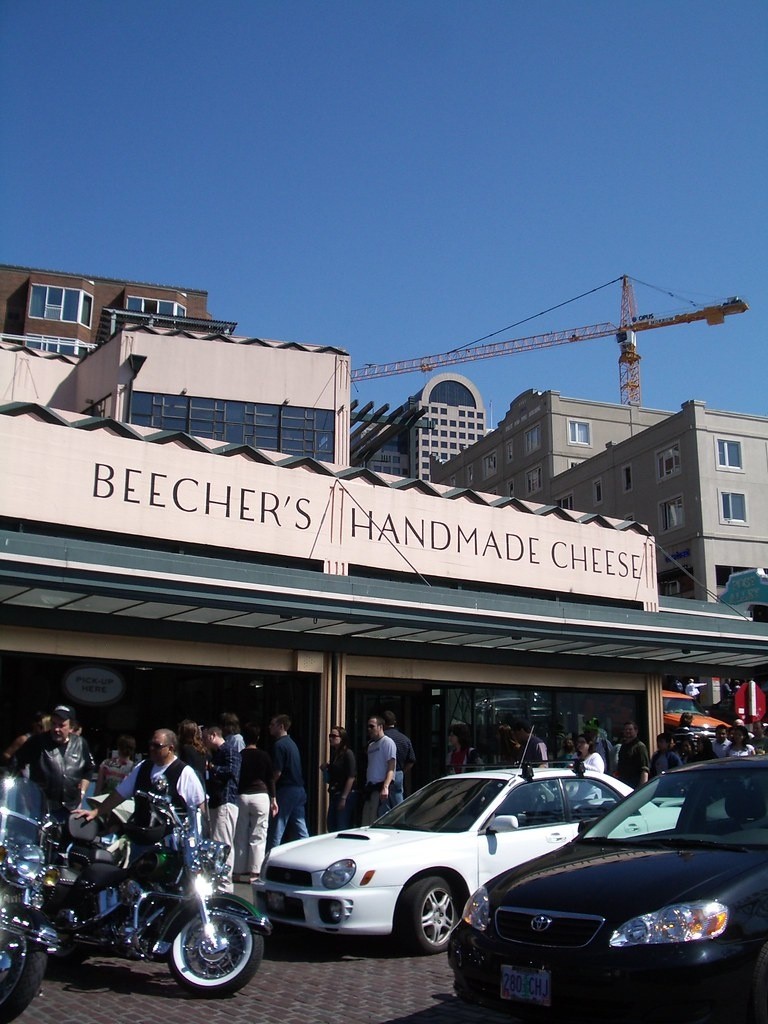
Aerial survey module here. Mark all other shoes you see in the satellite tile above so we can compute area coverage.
[232,876,240,883]
[248,877,259,886]
[111,947,144,961]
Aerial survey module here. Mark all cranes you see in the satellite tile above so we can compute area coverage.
[349,272,750,405]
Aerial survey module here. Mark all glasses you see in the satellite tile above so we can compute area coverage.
[329,734,340,739]
[575,740,585,744]
[149,740,167,750]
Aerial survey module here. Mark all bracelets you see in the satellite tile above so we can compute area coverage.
[80,790,85,795]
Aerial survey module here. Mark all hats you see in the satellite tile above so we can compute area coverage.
[49,705,75,720]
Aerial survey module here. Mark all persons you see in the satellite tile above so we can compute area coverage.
[444,724,486,775]
[2,704,416,947]
[500,677,768,789]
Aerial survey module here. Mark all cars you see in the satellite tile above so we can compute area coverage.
[442,755,768,1024]
[249,764,690,956]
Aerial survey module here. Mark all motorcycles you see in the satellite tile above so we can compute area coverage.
[0,770,90,1024]
[43,775,273,998]
